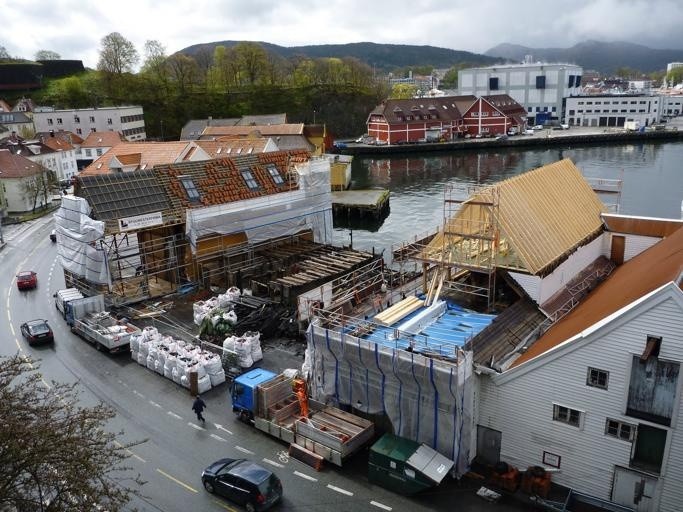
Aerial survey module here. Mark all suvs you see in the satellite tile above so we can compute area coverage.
[15,270,38,291]
[201,456,284,512]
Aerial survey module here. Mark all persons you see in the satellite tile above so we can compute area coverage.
[192,395,207,423]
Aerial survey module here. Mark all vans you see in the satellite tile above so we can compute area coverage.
[52,286,85,320]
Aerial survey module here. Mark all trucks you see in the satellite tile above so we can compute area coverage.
[229,367,376,468]
[64,296,142,360]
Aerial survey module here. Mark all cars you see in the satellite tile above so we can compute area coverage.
[19,319,55,347]
[49,228,57,243]
[352,122,571,145]
